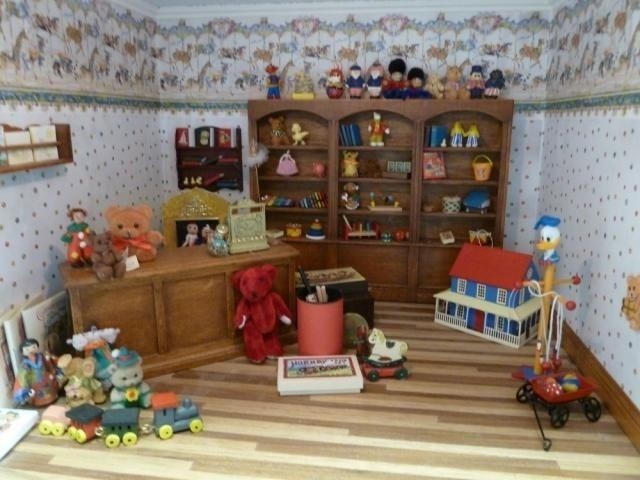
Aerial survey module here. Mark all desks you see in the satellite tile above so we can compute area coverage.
[57,236,300,379]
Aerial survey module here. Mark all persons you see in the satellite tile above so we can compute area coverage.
[450,121,480,147]
[368,111,390,147]
[264,57,507,100]
[59,207,100,269]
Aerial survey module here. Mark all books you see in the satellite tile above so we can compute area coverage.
[174,125,241,189]
[423,152,446,178]
[339,124,364,147]
[5,130,34,167]
[426,124,449,147]
[29,124,60,162]
[0,125,9,167]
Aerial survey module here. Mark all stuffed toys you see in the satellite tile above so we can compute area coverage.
[229,262,295,363]
[104,207,163,263]
[94,236,126,284]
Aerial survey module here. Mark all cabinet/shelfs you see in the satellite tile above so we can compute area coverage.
[0,121,74,174]
[175,126,244,192]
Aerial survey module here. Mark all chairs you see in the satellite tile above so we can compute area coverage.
[161,186,230,248]
